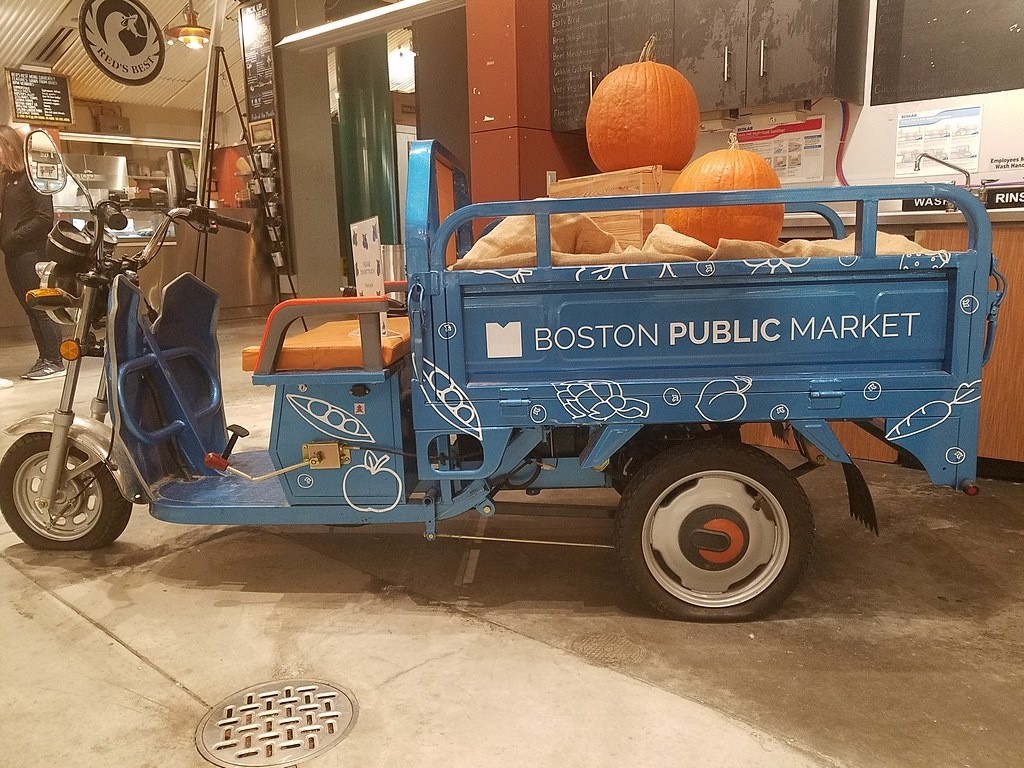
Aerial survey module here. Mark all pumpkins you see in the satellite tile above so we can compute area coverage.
[586,35,701,170]
[663,133,784,249]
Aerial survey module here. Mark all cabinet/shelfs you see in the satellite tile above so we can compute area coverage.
[700,219,1024,485]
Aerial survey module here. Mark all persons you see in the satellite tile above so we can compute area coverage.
[0,125,67,380]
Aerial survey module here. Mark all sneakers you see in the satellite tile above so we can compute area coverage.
[18,358,67,380]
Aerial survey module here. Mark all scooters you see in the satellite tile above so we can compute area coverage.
[0,128,1008,623]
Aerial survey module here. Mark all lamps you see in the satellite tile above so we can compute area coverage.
[163,0,211,50]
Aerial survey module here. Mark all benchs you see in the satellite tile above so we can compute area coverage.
[243,280,413,384]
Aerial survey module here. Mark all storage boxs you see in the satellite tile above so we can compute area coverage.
[548,166,682,251]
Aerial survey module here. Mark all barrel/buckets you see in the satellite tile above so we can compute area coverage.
[246,142,285,267]
[381,245,405,310]
